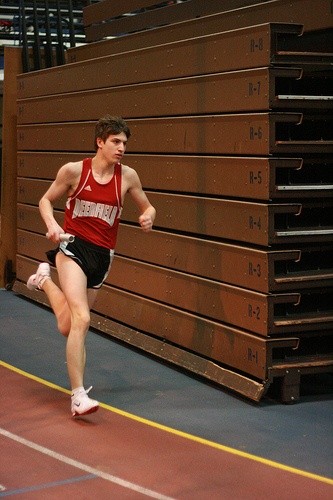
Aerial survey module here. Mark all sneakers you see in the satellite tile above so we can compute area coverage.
[71,391,100,417]
[27,262,51,290]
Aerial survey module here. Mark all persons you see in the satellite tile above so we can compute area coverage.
[25,116,158,418]
